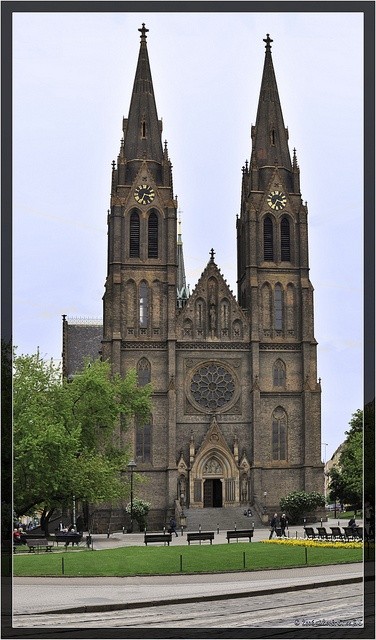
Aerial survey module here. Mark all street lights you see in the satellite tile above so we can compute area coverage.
[126,461,136,533]
[178,491,185,518]
[318,442,328,506]
[260,491,269,515]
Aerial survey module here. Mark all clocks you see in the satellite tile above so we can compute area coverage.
[267,191,287,210]
[134,184,155,204]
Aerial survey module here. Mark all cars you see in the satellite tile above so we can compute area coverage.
[325,503,341,511]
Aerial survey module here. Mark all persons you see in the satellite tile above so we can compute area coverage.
[168,517,178,537]
[280,513,288,537]
[268,512,279,540]
[348,518,359,528]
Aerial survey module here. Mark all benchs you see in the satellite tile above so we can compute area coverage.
[354,527,369,538]
[226,530,253,543]
[14,537,26,544]
[56,535,83,547]
[13,545,17,554]
[330,527,345,539]
[342,527,358,539]
[144,535,172,546]
[186,532,214,545]
[316,527,332,540]
[26,538,53,552]
[304,528,319,539]
